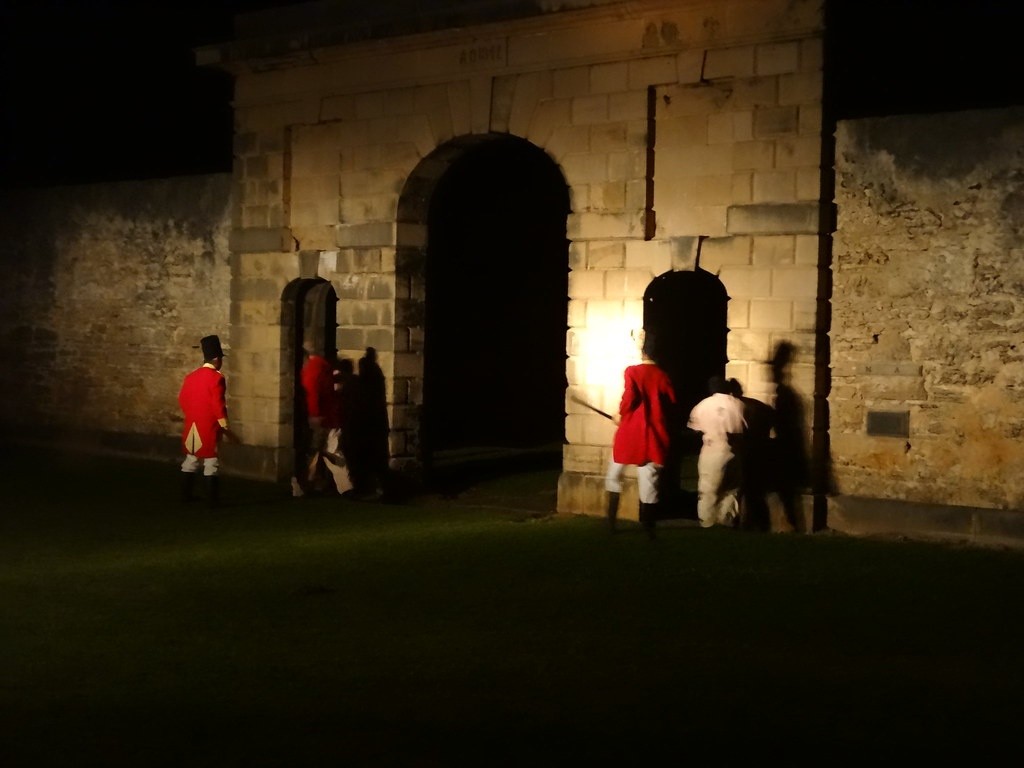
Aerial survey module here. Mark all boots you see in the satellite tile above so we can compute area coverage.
[180,470,198,504]
[605,492,619,536]
[639,501,656,542]
[205,474,228,510]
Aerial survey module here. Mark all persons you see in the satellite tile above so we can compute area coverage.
[291,328,359,499]
[605,341,678,541]
[682,375,751,530]
[178,335,232,507]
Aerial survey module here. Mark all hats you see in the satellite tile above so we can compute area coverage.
[200,336,228,359]
